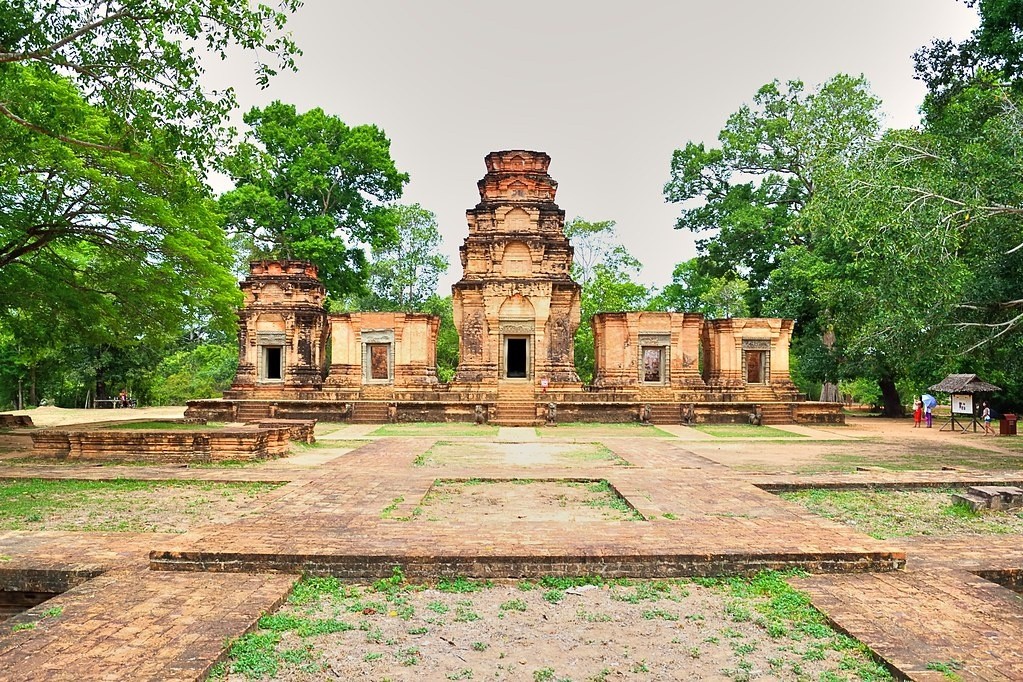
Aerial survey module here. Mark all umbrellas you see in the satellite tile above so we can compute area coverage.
[921,394,936,408]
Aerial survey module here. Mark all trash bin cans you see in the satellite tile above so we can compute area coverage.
[999,413,1018,437]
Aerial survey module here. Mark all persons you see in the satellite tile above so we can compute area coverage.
[981,401,997,437]
[924,404,932,428]
[912,395,923,428]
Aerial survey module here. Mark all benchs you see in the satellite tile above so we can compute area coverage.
[93,400,121,409]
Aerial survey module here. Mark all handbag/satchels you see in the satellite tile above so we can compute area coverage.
[924,416,929,422]
